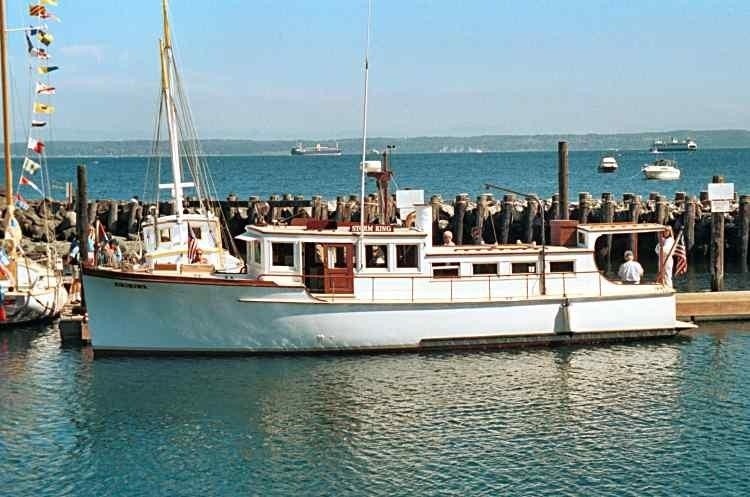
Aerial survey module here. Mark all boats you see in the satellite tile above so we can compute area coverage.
[290,139,344,156]
[598,155,619,170]
[647,133,698,152]
[640,160,681,180]
[64,0,699,357]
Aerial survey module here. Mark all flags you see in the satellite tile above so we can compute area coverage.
[0,0,59,287]
[187,222,202,264]
[674,232,688,278]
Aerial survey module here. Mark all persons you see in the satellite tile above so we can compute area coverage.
[371,244,385,264]
[59,218,124,306]
[594,242,610,277]
[441,230,458,246]
[654,223,675,288]
[191,247,209,264]
[616,250,644,286]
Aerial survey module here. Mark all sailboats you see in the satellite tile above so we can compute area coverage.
[1,0,70,329]
[129,0,247,273]
